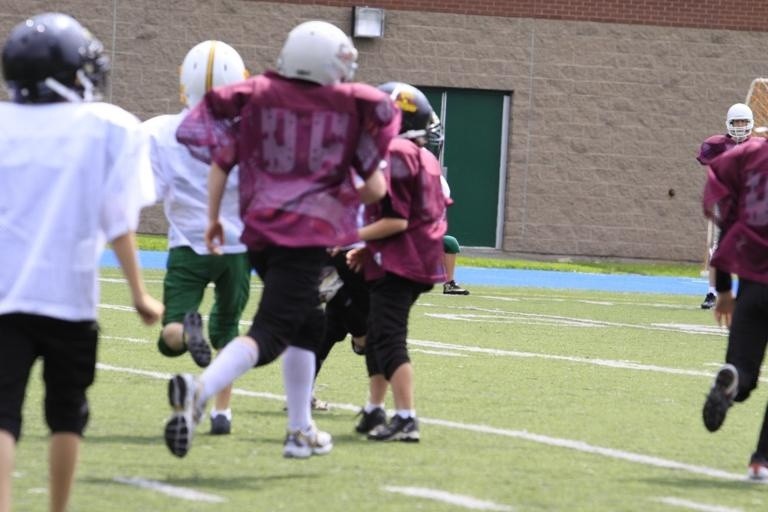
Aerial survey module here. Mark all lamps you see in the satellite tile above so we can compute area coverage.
[348,6,387,40]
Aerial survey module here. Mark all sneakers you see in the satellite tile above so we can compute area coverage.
[367,414,419,442]
[281,421,333,459]
[443,280,469,295]
[355,407,386,432]
[164,374,207,458]
[702,293,718,308]
[702,363,739,433]
[185,312,210,368]
[748,453,768,483]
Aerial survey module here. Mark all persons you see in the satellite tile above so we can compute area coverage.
[698,102,759,310]
[140,20,467,460]
[697,129,768,480]
[0,13,167,512]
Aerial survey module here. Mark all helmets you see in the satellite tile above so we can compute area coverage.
[177,40,250,111]
[275,21,358,86]
[1,12,111,104]
[725,103,754,143]
[374,82,432,139]
